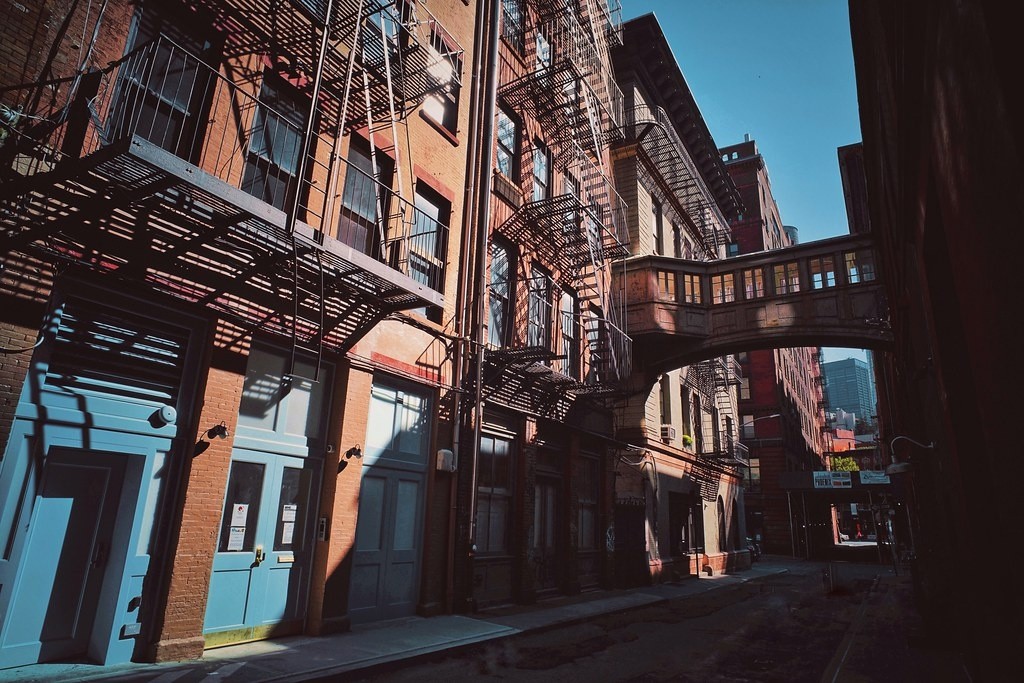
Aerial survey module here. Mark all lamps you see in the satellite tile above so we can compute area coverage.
[216,421,229,439]
[352,443,361,459]
[884,433,938,477]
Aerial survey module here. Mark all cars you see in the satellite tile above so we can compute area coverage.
[746,537,760,562]
[840,532,850,541]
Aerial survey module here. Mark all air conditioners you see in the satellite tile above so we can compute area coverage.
[662,426,675,439]
